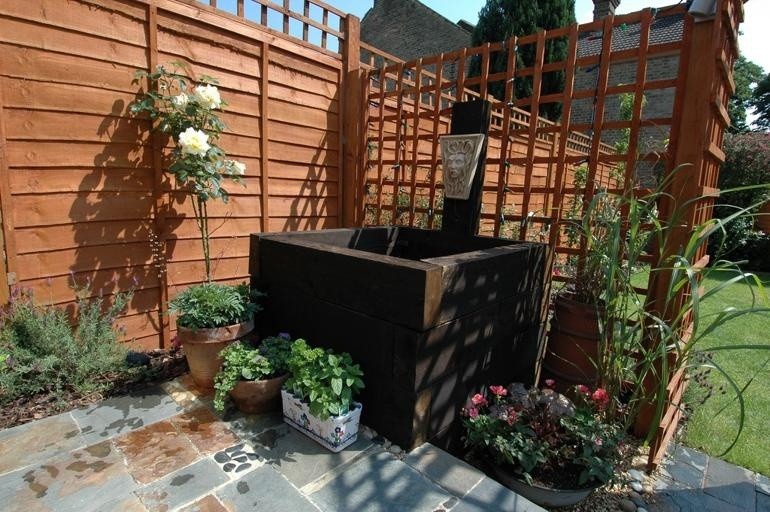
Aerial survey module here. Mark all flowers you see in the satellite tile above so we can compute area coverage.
[128,59,249,279]
[460,381,630,482]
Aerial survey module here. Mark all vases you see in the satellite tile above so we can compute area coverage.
[500,465,594,506]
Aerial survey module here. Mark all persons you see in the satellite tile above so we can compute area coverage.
[445,140,473,179]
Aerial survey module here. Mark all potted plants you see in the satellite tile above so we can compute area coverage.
[549,167,632,377]
[164,279,364,455]
